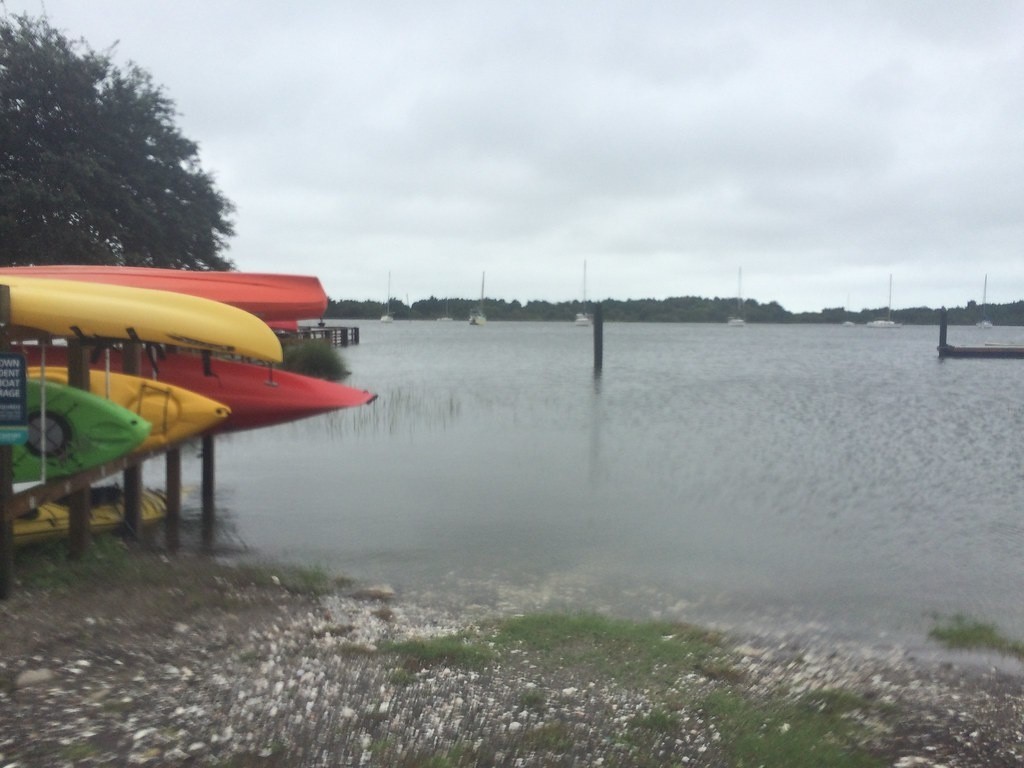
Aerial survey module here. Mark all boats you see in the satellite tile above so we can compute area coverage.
[1,376,158,486]
[0,265,329,323]
[1,343,380,437]
[0,275,284,367]
[12,485,173,542]
[21,365,231,455]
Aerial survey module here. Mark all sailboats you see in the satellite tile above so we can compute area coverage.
[864,274,903,329]
[575,258,589,326]
[729,265,745,327]
[469,272,487,325]
[437,300,454,321]
[976,275,993,328]
[381,270,395,323]
